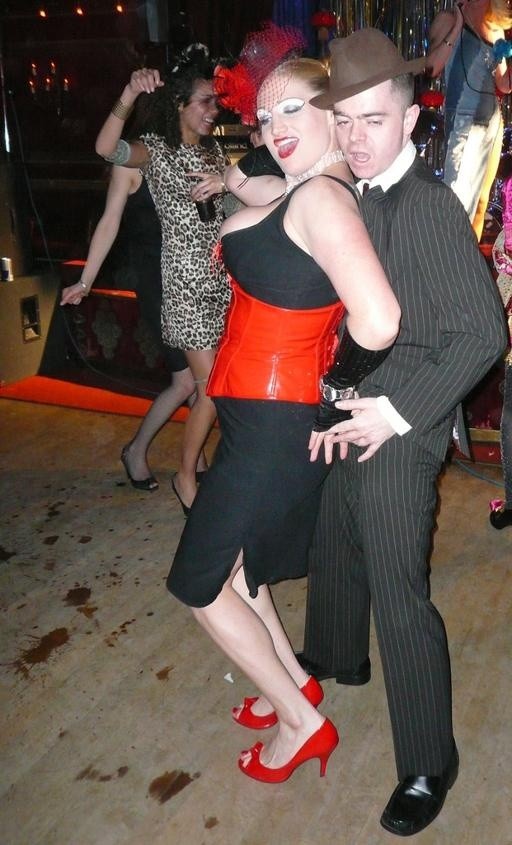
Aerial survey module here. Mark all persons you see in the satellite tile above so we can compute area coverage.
[289,27,512,838]
[163,25,402,782]
[429,1,511,242]
[61,42,247,516]
[489,177,512,529]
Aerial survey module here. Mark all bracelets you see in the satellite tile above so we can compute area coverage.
[443,40,453,47]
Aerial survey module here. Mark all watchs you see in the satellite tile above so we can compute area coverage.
[319,374,355,401]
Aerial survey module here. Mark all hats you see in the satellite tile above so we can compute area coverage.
[308,5,463,111]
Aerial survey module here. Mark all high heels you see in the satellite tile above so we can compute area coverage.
[120,445,159,492]
[171,470,211,518]
[231,675,340,784]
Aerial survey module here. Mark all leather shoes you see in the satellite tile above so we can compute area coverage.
[379,743,459,837]
[293,651,371,687]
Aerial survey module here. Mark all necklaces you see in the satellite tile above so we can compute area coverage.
[284,151,346,196]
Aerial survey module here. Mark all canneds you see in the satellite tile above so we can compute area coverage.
[1,257,13,281]
